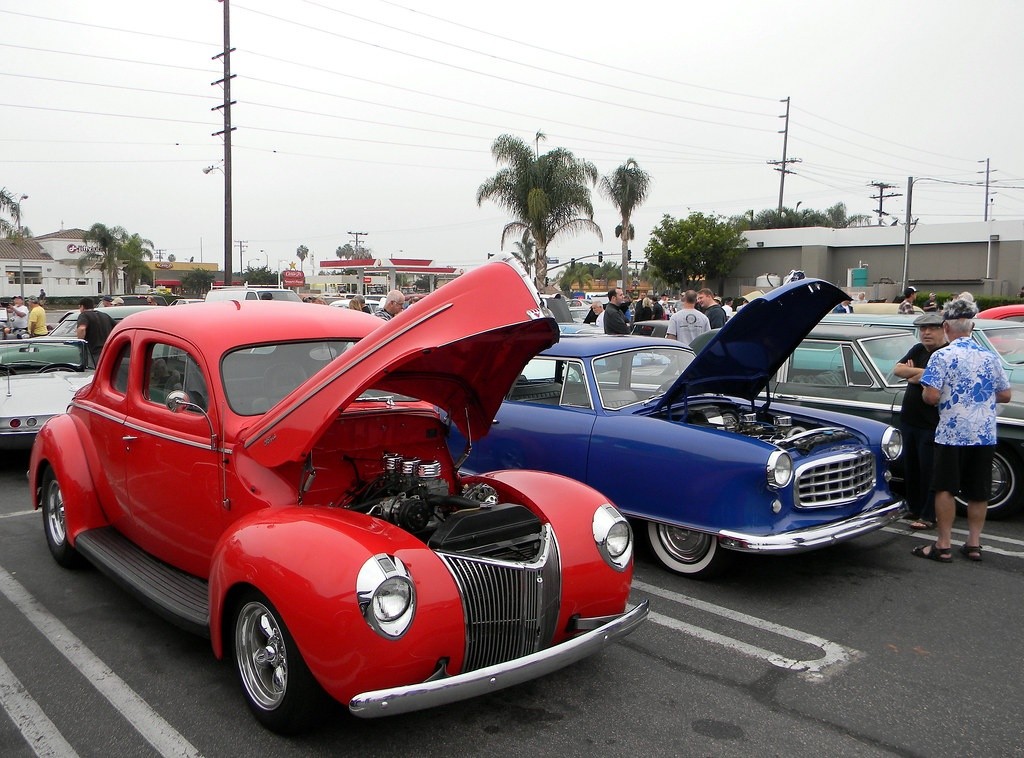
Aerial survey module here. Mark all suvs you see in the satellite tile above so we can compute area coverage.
[538,293,574,323]
[204,280,308,352]
[94,293,184,307]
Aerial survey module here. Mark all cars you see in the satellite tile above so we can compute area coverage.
[790,313,1024,386]
[554,322,670,372]
[25,251,651,736]
[573,291,610,309]
[973,304,1024,355]
[428,268,909,583]
[330,299,381,315]
[630,320,670,337]
[170,298,204,307]
[593,324,1024,522]
[565,299,595,319]
[0,339,100,451]
[46,305,171,389]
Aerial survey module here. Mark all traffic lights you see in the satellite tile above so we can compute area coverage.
[488,252,494,259]
[628,249,631,261]
[644,262,649,270]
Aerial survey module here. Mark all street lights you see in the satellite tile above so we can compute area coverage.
[203,166,233,286]
[261,249,270,271]
[247,257,260,271]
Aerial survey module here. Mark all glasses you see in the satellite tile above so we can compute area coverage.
[392,300,404,306]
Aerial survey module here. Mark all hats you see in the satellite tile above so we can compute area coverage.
[100,296,111,301]
[913,314,943,327]
[13,295,23,301]
[943,298,977,319]
[110,298,124,305]
[904,287,919,296]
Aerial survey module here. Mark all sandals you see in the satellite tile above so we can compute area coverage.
[911,541,953,563]
[959,544,983,560]
[910,519,937,529]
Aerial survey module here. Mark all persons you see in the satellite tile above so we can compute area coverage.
[76,296,124,366]
[893,298,1011,562]
[0,289,49,338]
[259,289,421,322]
[834,285,1024,319]
[146,296,159,306]
[590,286,748,371]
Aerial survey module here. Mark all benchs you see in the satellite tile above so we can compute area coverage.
[0,348,81,367]
[511,380,566,403]
[516,390,640,427]
[794,371,848,389]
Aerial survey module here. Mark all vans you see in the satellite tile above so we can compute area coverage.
[344,294,388,302]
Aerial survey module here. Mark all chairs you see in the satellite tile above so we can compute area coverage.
[255,363,308,415]
